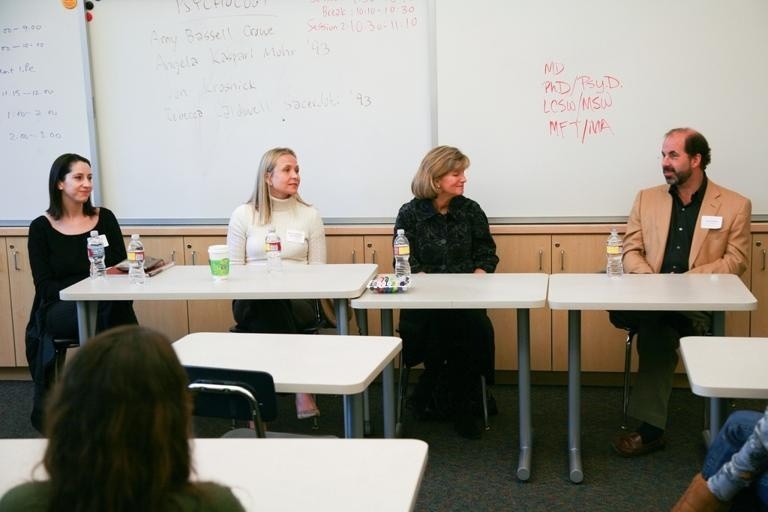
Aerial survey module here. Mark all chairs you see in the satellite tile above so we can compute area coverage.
[228,297,321,430]
[179,366,276,438]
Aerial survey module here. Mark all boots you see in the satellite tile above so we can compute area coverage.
[672,473,733,512]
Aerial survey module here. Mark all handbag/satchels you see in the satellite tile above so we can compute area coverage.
[116,255,165,271]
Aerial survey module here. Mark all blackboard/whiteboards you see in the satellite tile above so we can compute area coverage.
[80,0,437,227]
[431,0,768,223]
[0,0,103,227]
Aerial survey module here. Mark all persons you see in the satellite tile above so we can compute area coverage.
[609,127,752,457]
[0,325,250,512]
[670,403,768,512]
[25,153,139,439]
[390,145,499,418]
[226,147,328,420]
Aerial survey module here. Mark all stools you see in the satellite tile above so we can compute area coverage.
[620,321,713,431]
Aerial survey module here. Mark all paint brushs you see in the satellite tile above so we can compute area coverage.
[371,274,410,293]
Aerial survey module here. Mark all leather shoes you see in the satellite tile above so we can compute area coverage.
[295,400,320,419]
[613,433,665,457]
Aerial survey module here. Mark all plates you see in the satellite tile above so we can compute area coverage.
[115,257,163,273]
[367,275,416,295]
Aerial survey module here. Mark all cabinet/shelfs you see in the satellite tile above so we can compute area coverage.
[1,230,35,380]
[122,230,234,342]
[325,230,410,384]
[488,227,638,385]
[725,223,768,338]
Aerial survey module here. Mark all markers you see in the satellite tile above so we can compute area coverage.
[148,262,176,276]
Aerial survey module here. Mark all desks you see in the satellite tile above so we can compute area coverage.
[0,438,429,512]
[351,273,548,480]
[58,262,380,334]
[549,273,759,482]
[171,331,404,438]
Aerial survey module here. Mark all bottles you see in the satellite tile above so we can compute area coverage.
[87,231,107,281]
[606,228,623,282]
[126,233,145,289]
[265,228,283,276]
[392,229,411,279]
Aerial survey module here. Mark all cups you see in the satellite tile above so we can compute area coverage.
[207,244,231,281]
